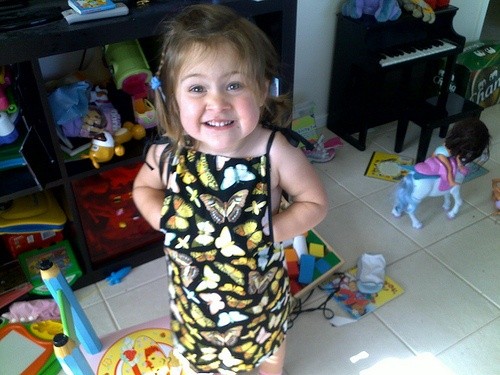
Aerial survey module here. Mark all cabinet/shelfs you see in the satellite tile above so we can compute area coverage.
[0,0,296,315]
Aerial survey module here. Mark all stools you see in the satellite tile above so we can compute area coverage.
[393,92,484,165]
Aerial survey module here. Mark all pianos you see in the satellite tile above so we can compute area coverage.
[326,7,467,152]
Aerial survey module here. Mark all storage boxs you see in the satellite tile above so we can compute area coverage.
[438,39,499,112]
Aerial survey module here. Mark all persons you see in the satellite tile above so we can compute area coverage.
[133,5,328,375]
